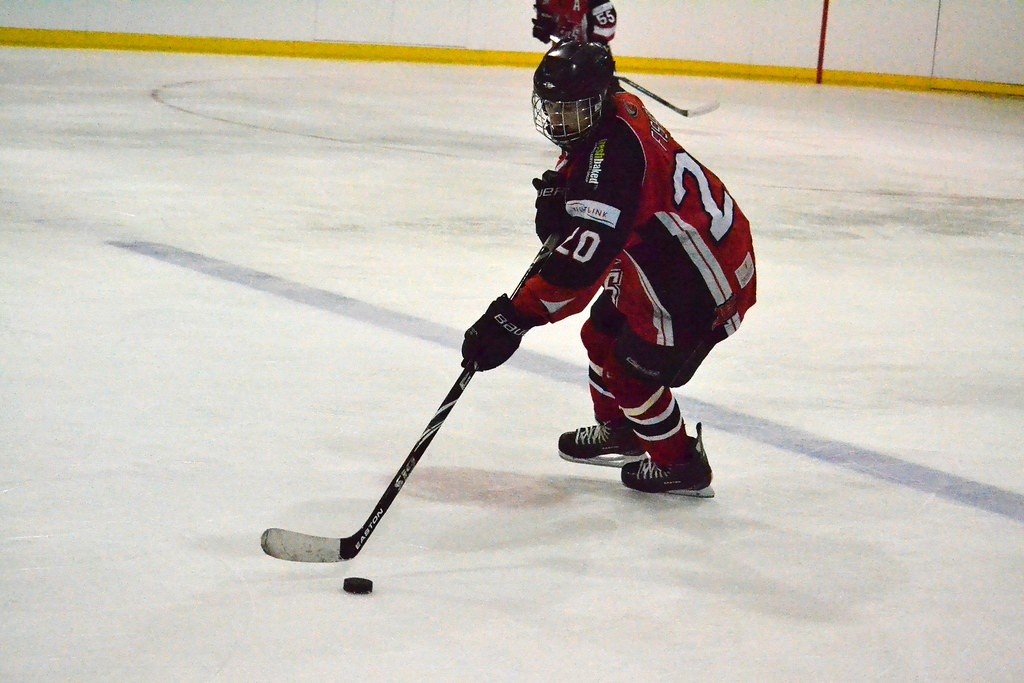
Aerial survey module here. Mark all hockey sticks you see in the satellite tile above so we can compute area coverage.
[547,33,720,119]
[260,228,561,563]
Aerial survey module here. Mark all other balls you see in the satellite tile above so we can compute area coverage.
[341,574,376,596]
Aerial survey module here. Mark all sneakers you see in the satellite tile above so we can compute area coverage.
[557,418,648,467]
[621,450,713,498]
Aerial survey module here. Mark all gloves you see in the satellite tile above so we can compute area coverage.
[532,178,570,245]
[533,16,556,43]
[462,292,535,371]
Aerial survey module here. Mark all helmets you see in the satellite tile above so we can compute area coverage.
[533,38,614,94]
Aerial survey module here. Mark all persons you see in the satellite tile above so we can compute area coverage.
[461,35,757,499]
[532,0,616,52]
